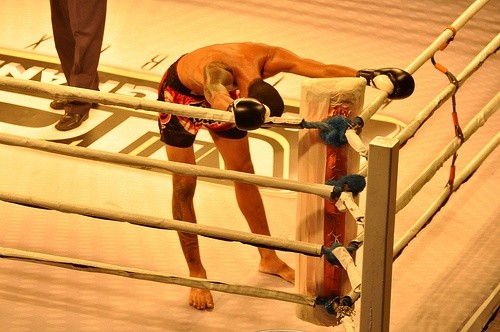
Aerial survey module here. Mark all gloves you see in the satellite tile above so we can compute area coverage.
[227,97,270,131]
[356,67,415,100]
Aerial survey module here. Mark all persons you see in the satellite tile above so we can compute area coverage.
[158,43,415,310]
[50,0,107,131]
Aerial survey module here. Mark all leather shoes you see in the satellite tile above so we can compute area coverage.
[50,99,97,109]
[55,113,89,131]
